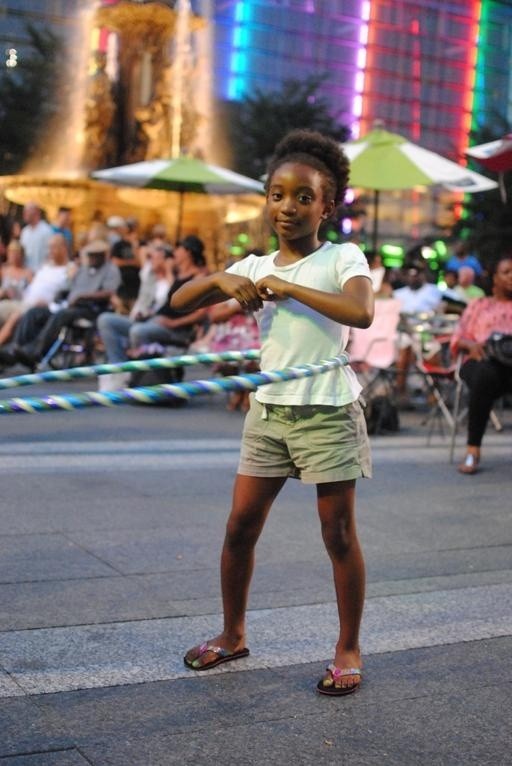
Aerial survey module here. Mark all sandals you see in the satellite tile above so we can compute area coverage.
[460,451,480,474]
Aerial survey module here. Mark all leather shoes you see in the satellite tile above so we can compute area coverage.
[2,345,37,369]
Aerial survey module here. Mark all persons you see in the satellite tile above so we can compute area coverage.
[169,128,376,697]
[348,241,512,474]
[1,206,261,412]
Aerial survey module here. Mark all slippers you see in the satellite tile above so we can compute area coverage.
[184,637,249,671]
[317,664,362,695]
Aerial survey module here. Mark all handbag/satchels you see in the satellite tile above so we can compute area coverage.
[485,333,512,367]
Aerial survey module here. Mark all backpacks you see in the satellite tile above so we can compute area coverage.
[132,354,186,408]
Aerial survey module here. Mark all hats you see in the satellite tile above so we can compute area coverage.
[175,235,206,267]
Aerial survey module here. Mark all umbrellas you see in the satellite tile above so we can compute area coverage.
[259,118,499,250]
[89,148,267,247]
